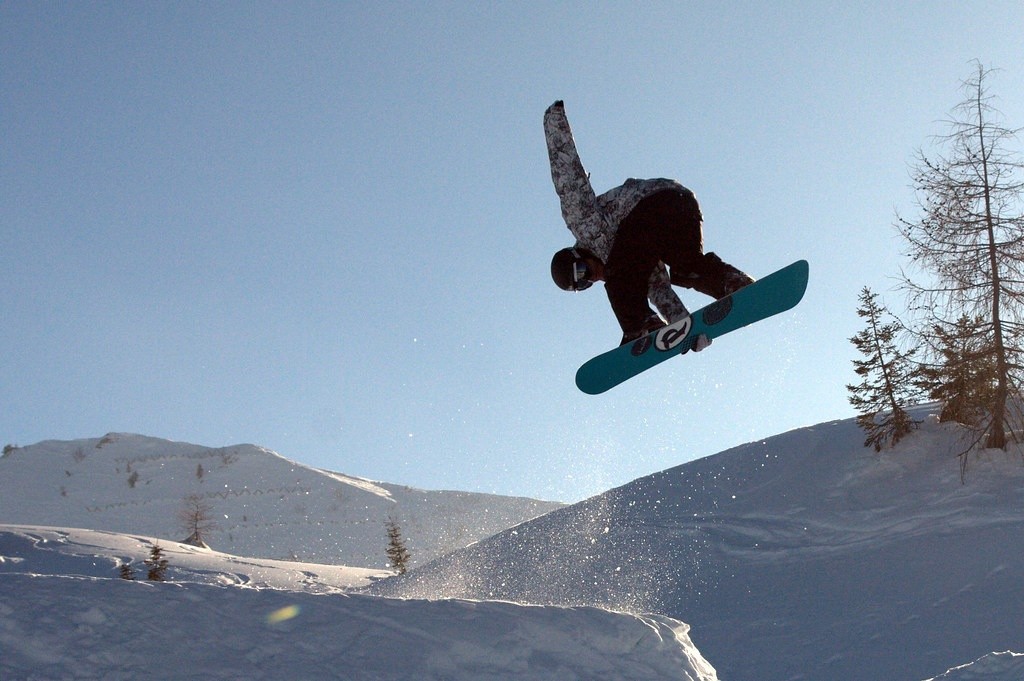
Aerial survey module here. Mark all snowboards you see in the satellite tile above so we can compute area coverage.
[574,257,810,396]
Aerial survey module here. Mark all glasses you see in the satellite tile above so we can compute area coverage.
[572,256,594,292]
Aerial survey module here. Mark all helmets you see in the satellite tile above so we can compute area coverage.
[550,247,600,293]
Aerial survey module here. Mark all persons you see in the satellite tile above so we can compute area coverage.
[544,99,755,354]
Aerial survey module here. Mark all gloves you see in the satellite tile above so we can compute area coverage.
[681,333,713,355]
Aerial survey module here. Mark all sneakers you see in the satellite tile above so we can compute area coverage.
[619,313,666,349]
[717,272,755,299]
